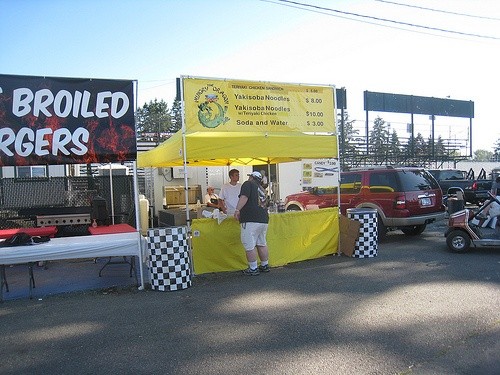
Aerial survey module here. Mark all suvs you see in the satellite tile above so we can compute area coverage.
[285,166,446,242]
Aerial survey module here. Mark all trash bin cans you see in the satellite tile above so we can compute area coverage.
[447,198,465,215]
[146,225,193,292]
[345,207,378,259]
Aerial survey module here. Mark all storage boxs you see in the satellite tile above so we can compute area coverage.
[163,185,201,205]
[157,208,198,228]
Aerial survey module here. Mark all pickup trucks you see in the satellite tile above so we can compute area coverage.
[426,169,497,205]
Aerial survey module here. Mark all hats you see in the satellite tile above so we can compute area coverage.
[207,186,215,190]
[247,171,262,182]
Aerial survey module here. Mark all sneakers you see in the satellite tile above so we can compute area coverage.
[257,264,270,272]
[242,267,260,275]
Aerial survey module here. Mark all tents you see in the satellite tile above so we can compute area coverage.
[137,124,340,212]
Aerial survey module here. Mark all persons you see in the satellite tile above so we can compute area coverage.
[203,185,224,210]
[218,169,242,216]
[474,196,500,228]
[259,169,267,184]
[233,171,270,275]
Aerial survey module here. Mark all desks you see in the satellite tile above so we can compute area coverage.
[0,226,58,300]
[88,223,137,278]
[0,230,148,301]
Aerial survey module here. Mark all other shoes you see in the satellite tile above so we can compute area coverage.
[475,213,488,220]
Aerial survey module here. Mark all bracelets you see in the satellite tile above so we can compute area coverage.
[234,207,240,213]
